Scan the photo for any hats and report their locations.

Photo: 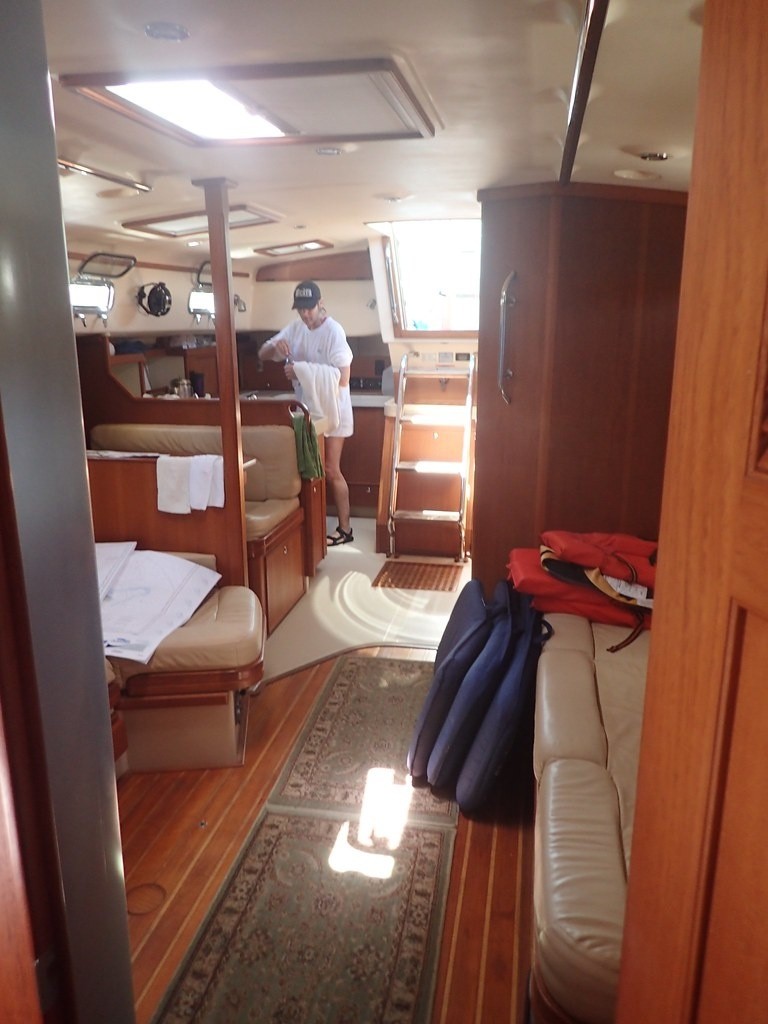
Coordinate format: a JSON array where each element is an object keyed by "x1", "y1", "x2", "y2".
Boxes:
[{"x1": 291, "y1": 280, "x2": 321, "y2": 310}]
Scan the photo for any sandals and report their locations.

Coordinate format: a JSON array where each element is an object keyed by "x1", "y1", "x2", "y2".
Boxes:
[{"x1": 326, "y1": 526, "x2": 354, "y2": 546}]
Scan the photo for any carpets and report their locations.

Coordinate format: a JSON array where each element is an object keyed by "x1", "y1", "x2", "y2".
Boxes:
[{"x1": 151, "y1": 654, "x2": 480, "y2": 1024}]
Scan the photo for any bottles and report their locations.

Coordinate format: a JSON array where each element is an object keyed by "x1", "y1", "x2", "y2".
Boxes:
[{"x1": 285, "y1": 355, "x2": 294, "y2": 365}]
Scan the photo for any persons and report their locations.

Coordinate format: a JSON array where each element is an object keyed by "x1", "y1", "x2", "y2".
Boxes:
[{"x1": 257, "y1": 281, "x2": 353, "y2": 547}]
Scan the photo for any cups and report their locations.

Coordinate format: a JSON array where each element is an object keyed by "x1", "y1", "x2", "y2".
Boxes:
[{"x1": 178, "y1": 379, "x2": 191, "y2": 398}]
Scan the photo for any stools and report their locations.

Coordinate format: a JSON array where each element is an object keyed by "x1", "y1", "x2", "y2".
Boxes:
[{"x1": 93, "y1": 552, "x2": 266, "y2": 767}]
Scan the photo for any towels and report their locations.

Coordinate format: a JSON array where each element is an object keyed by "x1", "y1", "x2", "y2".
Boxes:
[
  {"x1": 290, "y1": 414, "x2": 326, "y2": 481},
  {"x1": 156, "y1": 455, "x2": 225, "y2": 515},
  {"x1": 292, "y1": 360, "x2": 341, "y2": 437},
  {"x1": 95, "y1": 542, "x2": 223, "y2": 665}
]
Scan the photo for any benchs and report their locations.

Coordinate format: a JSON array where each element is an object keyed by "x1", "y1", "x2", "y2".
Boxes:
[
  {"x1": 519, "y1": 604, "x2": 648, "y2": 1024},
  {"x1": 88, "y1": 422, "x2": 306, "y2": 640}
]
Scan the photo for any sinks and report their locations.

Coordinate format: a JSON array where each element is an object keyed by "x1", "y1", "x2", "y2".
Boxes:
[{"x1": 293, "y1": 414, "x2": 323, "y2": 424}]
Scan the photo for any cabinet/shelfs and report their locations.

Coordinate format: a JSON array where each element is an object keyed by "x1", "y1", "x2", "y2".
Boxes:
[{"x1": 324, "y1": 406, "x2": 384, "y2": 506}]
